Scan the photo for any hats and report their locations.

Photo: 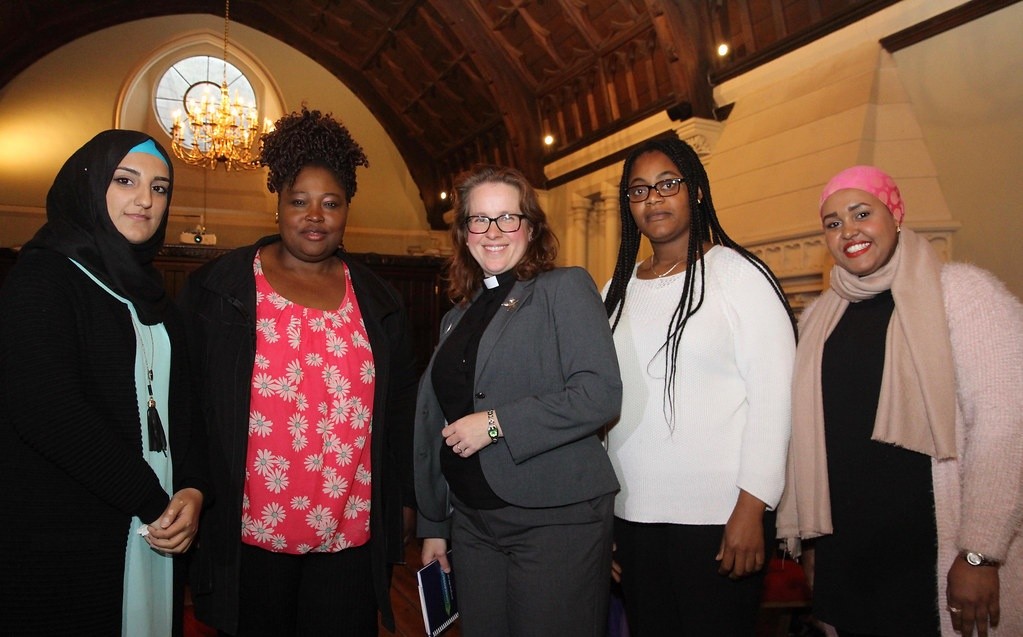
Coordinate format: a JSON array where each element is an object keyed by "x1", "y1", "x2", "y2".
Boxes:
[{"x1": 820, "y1": 165, "x2": 909, "y2": 223}]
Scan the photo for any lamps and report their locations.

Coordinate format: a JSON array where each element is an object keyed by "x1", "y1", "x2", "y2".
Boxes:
[{"x1": 169, "y1": 1, "x2": 276, "y2": 174}]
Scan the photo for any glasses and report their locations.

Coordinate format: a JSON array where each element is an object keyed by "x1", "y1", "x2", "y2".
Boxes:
[
  {"x1": 466, "y1": 214, "x2": 528, "y2": 235},
  {"x1": 623, "y1": 178, "x2": 689, "y2": 203}
]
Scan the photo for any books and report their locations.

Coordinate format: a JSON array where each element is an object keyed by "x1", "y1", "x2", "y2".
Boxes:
[{"x1": 416, "y1": 548, "x2": 460, "y2": 636}]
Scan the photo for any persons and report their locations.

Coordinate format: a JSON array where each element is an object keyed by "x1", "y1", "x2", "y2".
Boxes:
[
  {"x1": 0, "y1": 128, "x2": 216, "y2": 637},
  {"x1": 178, "y1": 102, "x2": 421, "y2": 635},
  {"x1": 598, "y1": 137, "x2": 796, "y2": 637},
  {"x1": 410, "y1": 164, "x2": 625, "y2": 636},
  {"x1": 793, "y1": 165, "x2": 1022, "y2": 637}
]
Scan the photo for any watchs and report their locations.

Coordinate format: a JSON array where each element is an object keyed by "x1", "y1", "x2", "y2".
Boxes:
[{"x1": 959, "y1": 549, "x2": 1001, "y2": 567}]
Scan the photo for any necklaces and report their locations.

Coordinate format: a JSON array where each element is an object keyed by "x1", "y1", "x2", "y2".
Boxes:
[
  {"x1": 647, "y1": 249, "x2": 689, "y2": 279},
  {"x1": 129, "y1": 303, "x2": 168, "y2": 455}
]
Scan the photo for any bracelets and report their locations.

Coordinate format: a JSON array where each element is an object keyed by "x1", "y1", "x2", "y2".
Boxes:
[{"x1": 486, "y1": 410, "x2": 498, "y2": 443}]
